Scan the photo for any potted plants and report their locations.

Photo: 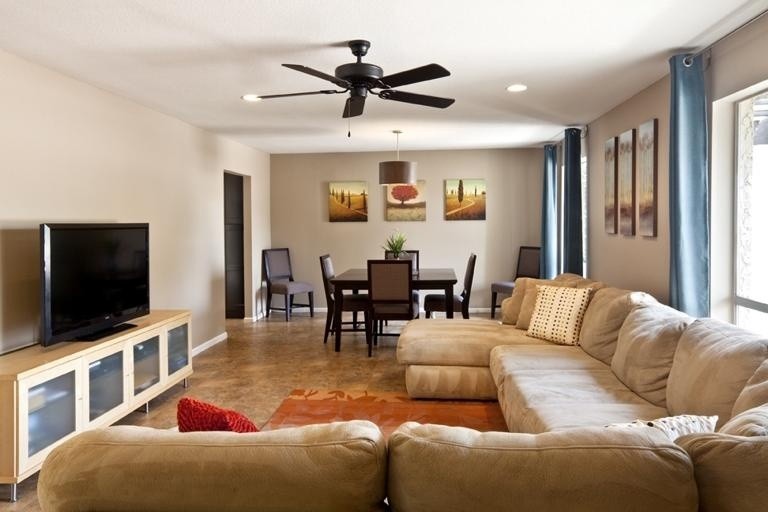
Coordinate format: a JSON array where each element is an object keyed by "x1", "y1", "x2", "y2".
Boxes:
[
  {"x1": 382, "y1": 226, "x2": 408, "y2": 260},
  {"x1": 490, "y1": 246, "x2": 540, "y2": 320}
]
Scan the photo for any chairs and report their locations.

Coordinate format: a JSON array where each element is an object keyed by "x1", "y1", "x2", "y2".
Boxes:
[
  {"x1": 319, "y1": 250, "x2": 477, "y2": 357},
  {"x1": 262, "y1": 248, "x2": 314, "y2": 322}
]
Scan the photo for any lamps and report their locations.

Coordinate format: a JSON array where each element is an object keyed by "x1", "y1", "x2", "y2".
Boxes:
[{"x1": 378, "y1": 130, "x2": 416, "y2": 186}]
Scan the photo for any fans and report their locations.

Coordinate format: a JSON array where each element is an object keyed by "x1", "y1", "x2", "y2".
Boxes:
[{"x1": 257, "y1": 40, "x2": 455, "y2": 119}]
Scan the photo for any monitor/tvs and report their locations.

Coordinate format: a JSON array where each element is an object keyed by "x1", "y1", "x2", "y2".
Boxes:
[{"x1": 40, "y1": 224, "x2": 149, "y2": 346}]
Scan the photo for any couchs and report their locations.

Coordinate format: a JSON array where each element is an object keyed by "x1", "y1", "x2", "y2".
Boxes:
[
  {"x1": 37, "y1": 424, "x2": 768, "y2": 512},
  {"x1": 397, "y1": 273, "x2": 611, "y2": 398},
  {"x1": 489, "y1": 287, "x2": 768, "y2": 435}
]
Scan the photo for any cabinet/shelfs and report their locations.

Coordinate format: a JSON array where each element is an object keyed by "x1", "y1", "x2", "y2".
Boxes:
[{"x1": 0, "y1": 308, "x2": 193, "y2": 502}]
